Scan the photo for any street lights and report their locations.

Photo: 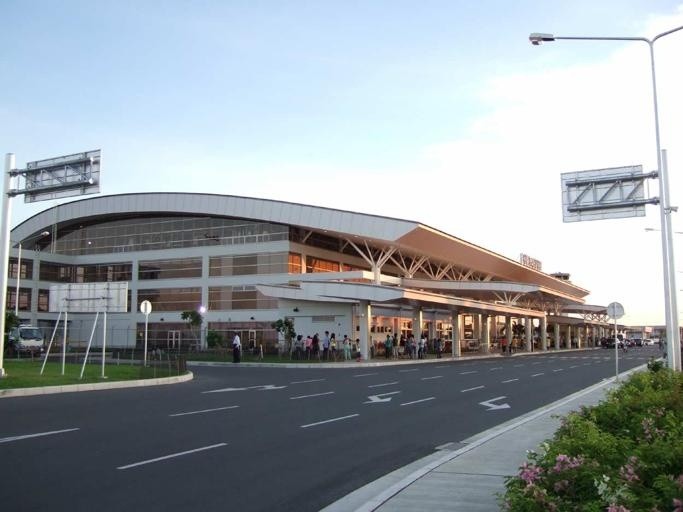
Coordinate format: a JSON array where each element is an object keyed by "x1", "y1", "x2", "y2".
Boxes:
[
  {"x1": 14, "y1": 231, "x2": 50, "y2": 319},
  {"x1": 529, "y1": 28, "x2": 676, "y2": 367}
]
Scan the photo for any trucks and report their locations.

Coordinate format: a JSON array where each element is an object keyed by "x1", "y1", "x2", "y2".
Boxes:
[{"x1": 5, "y1": 324, "x2": 44, "y2": 358}]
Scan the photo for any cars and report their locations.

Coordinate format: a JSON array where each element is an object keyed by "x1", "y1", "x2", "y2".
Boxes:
[{"x1": 600, "y1": 332, "x2": 661, "y2": 349}]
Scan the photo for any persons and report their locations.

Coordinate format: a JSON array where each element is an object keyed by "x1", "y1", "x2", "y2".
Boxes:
[
  {"x1": 384, "y1": 334, "x2": 392, "y2": 360},
  {"x1": 230, "y1": 330, "x2": 241, "y2": 364},
  {"x1": 623, "y1": 340, "x2": 628, "y2": 353},
  {"x1": 498, "y1": 336, "x2": 507, "y2": 353},
  {"x1": 354, "y1": 338, "x2": 362, "y2": 362},
  {"x1": 399, "y1": 334, "x2": 428, "y2": 360},
  {"x1": 391, "y1": 332, "x2": 399, "y2": 361},
  {"x1": 510, "y1": 335, "x2": 517, "y2": 353},
  {"x1": 435, "y1": 339, "x2": 442, "y2": 358},
  {"x1": 286, "y1": 330, "x2": 352, "y2": 362}
]
